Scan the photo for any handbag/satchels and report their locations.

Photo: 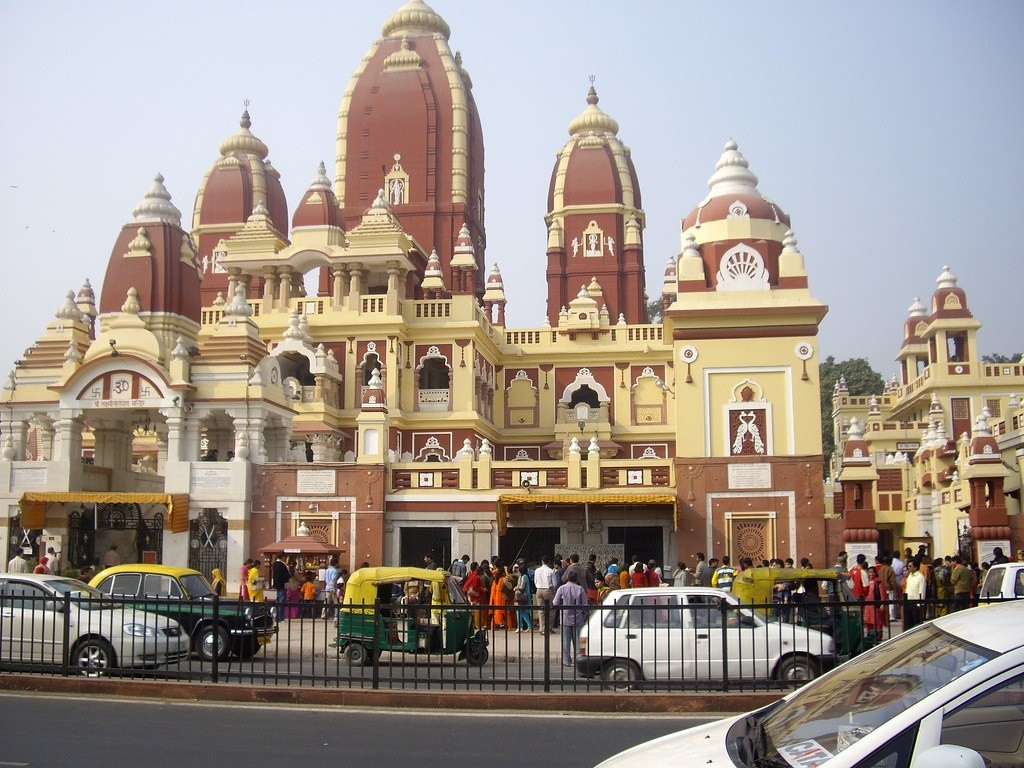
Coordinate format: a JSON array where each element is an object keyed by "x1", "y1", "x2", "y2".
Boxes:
[{"x1": 514, "y1": 589, "x2": 528, "y2": 602}]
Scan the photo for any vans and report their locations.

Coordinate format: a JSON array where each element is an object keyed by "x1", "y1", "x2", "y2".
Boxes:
[{"x1": 978, "y1": 562, "x2": 1024, "y2": 607}]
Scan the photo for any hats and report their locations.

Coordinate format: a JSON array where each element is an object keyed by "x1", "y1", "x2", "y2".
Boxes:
[{"x1": 40, "y1": 556, "x2": 48, "y2": 564}]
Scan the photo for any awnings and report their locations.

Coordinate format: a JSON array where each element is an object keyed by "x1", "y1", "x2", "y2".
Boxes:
[
  {"x1": 18, "y1": 491, "x2": 190, "y2": 533},
  {"x1": 494, "y1": 493, "x2": 681, "y2": 536}
]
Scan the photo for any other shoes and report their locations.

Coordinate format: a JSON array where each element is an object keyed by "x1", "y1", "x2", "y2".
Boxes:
[
  {"x1": 539, "y1": 631, "x2": 545, "y2": 635},
  {"x1": 548, "y1": 628, "x2": 556, "y2": 633},
  {"x1": 524, "y1": 629, "x2": 532, "y2": 633},
  {"x1": 514, "y1": 628, "x2": 523, "y2": 633}
]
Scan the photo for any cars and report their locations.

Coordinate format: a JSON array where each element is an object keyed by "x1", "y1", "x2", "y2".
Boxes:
[
  {"x1": 0, "y1": 571, "x2": 192, "y2": 677},
  {"x1": 570, "y1": 586, "x2": 836, "y2": 692},
  {"x1": 591, "y1": 599, "x2": 1024, "y2": 768},
  {"x1": 45, "y1": 563, "x2": 280, "y2": 660}
]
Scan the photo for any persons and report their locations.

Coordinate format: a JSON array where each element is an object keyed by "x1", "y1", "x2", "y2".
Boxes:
[
  {"x1": 604, "y1": 235, "x2": 615, "y2": 256},
  {"x1": 589, "y1": 234, "x2": 600, "y2": 255},
  {"x1": 33, "y1": 547, "x2": 59, "y2": 575},
  {"x1": 102, "y1": 543, "x2": 121, "y2": 571},
  {"x1": 79, "y1": 566, "x2": 93, "y2": 583},
  {"x1": 226, "y1": 451, "x2": 234, "y2": 460},
  {"x1": 553, "y1": 570, "x2": 589, "y2": 667},
  {"x1": 571, "y1": 237, "x2": 583, "y2": 258},
  {"x1": 424, "y1": 545, "x2": 1012, "y2": 638},
  {"x1": 391, "y1": 178, "x2": 402, "y2": 205},
  {"x1": 211, "y1": 568, "x2": 226, "y2": 597},
  {"x1": 203, "y1": 449, "x2": 218, "y2": 461},
  {"x1": 202, "y1": 254, "x2": 212, "y2": 274},
  {"x1": 8, "y1": 547, "x2": 29, "y2": 573},
  {"x1": 239, "y1": 552, "x2": 370, "y2": 621}
]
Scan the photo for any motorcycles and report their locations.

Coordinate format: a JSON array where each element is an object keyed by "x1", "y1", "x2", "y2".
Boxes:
[
  {"x1": 328, "y1": 564, "x2": 490, "y2": 667},
  {"x1": 729, "y1": 567, "x2": 869, "y2": 657}
]
